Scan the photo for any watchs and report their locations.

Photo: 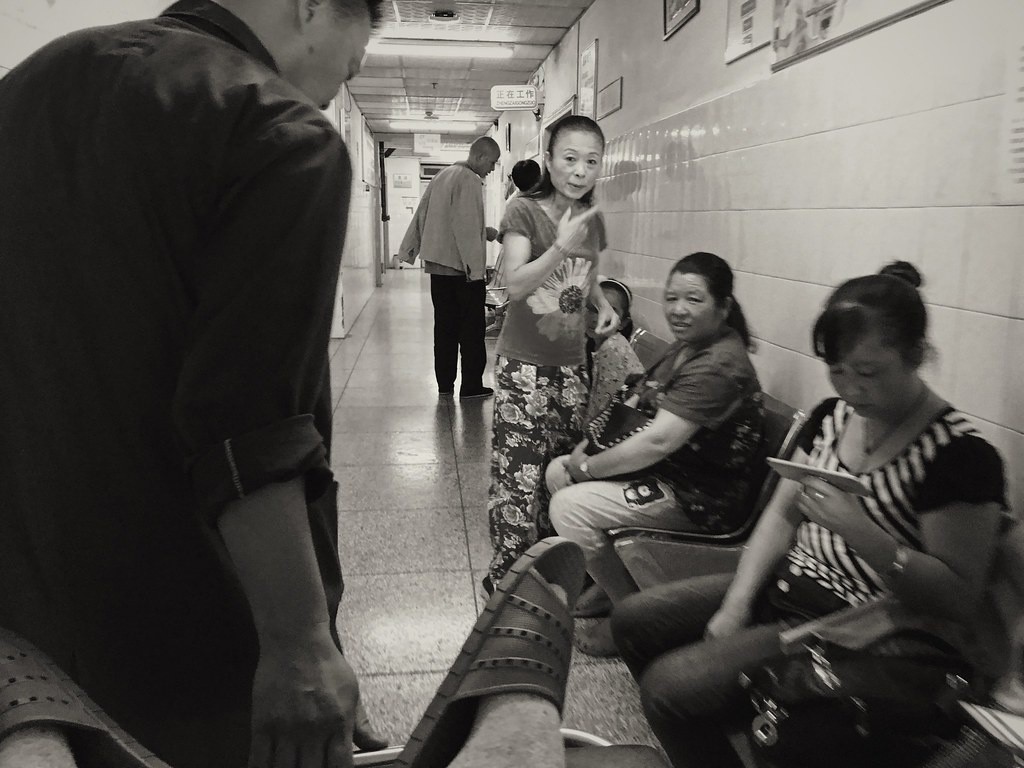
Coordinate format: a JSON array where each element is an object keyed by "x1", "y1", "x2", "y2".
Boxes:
[
  {"x1": 874, "y1": 539, "x2": 912, "y2": 581},
  {"x1": 581, "y1": 455, "x2": 595, "y2": 480}
]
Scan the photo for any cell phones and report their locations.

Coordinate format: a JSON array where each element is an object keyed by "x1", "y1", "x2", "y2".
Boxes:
[{"x1": 765, "y1": 456, "x2": 874, "y2": 497}]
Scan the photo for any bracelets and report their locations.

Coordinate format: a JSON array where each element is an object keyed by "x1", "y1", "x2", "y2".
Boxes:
[{"x1": 549, "y1": 239, "x2": 574, "y2": 259}]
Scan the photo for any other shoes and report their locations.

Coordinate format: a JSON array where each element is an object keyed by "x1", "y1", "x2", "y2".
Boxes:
[
  {"x1": 572, "y1": 618, "x2": 620, "y2": 659},
  {"x1": 571, "y1": 584, "x2": 614, "y2": 619},
  {"x1": 438, "y1": 388, "x2": 454, "y2": 394},
  {"x1": 460, "y1": 386, "x2": 493, "y2": 399}
]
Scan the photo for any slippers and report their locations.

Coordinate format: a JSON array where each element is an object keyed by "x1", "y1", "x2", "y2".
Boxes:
[
  {"x1": 394, "y1": 535, "x2": 586, "y2": 768},
  {"x1": 1, "y1": 625, "x2": 175, "y2": 768}
]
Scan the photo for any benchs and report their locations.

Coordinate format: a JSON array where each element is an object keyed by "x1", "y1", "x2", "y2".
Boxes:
[
  {"x1": 478, "y1": 247, "x2": 512, "y2": 334},
  {"x1": 612, "y1": 460, "x2": 1022, "y2": 768},
  {"x1": 526, "y1": 330, "x2": 805, "y2": 543}
]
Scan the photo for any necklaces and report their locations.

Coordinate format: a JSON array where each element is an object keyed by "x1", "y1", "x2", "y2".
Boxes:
[{"x1": 862, "y1": 380, "x2": 928, "y2": 457}]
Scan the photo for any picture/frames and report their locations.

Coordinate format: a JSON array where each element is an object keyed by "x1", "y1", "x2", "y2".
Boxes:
[
  {"x1": 576, "y1": 37, "x2": 598, "y2": 126},
  {"x1": 596, "y1": 77, "x2": 623, "y2": 123},
  {"x1": 722, "y1": 1, "x2": 774, "y2": 65},
  {"x1": 661, "y1": 1, "x2": 699, "y2": 42},
  {"x1": 769, "y1": 0, "x2": 952, "y2": 72}
]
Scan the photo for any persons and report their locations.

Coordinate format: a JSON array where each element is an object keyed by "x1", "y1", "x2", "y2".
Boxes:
[
  {"x1": 395, "y1": 136, "x2": 501, "y2": 399},
  {"x1": 496, "y1": 158, "x2": 541, "y2": 244},
  {"x1": 0, "y1": 0, "x2": 388, "y2": 768},
  {"x1": 481, "y1": 115, "x2": 624, "y2": 600},
  {"x1": 0, "y1": 535, "x2": 585, "y2": 767},
  {"x1": 581, "y1": 278, "x2": 648, "y2": 439},
  {"x1": 544, "y1": 251, "x2": 767, "y2": 658},
  {"x1": 606, "y1": 259, "x2": 1010, "y2": 767}
]
